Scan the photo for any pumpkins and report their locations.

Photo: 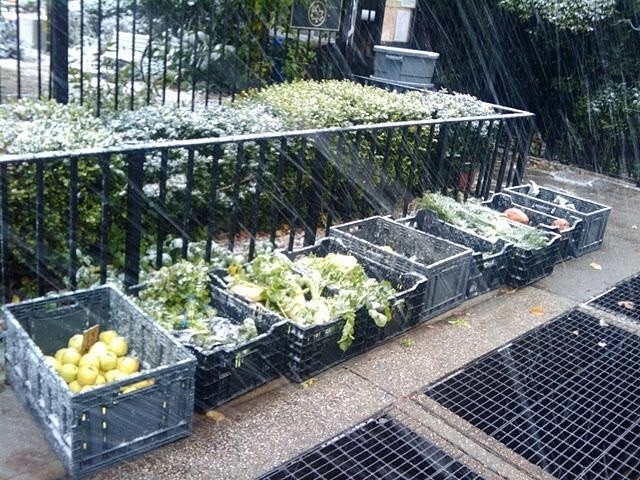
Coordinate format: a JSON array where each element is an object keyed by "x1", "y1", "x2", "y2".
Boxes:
[
  {"x1": 551, "y1": 218, "x2": 571, "y2": 230},
  {"x1": 504, "y1": 207, "x2": 529, "y2": 225}
]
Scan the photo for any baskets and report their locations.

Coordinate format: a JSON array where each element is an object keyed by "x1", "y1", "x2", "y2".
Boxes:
[
  {"x1": 329, "y1": 184, "x2": 612, "y2": 321},
  {"x1": 126, "y1": 279, "x2": 289, "y2": 414},
  {"x1": 0, "y1": 284, "x2": 196, "y2": 478},
  {"x1": 211, "y1": 237, "x2": 428, "y2": 383}
]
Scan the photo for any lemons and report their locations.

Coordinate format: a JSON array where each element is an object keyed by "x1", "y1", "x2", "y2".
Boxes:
[{"x1": 44, "y1": 330, "x2": 155, "y2": 396}]
